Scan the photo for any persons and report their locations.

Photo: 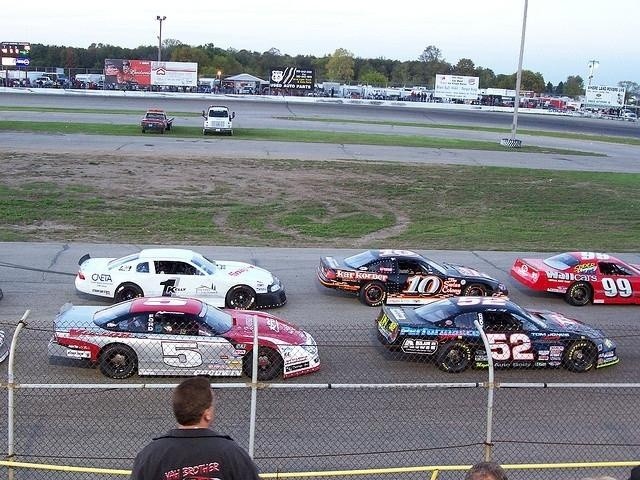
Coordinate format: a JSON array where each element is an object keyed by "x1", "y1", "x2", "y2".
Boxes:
[
  {"x1": 116, "y1": 60, "x2": 134, "y2": 83},
  {"x1": 476, "y1": 95, "x2": 500, "y2": 107},
  {"x1": 371, "y1": 88, "x2": 433, "y2": 103},
  {"x1": 464, "y1": 461, "x2": 508, "y2": 480},
  {"x1": 128, "y1": 377, "x2": 260, "y2": 480}
]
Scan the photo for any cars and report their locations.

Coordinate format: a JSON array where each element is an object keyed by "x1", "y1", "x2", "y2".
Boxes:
[
  {"x1": 0, "y1": 74, "x2": 103, "y2": 87},
  {"x1": 541, "y1": 104, "x2": 637, "y2": 121}
]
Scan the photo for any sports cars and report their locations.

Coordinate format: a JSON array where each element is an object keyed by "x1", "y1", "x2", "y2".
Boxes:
[
  {"x1": 75, "y1": 248, "x2": 287, "y2": 310},
  {"x1": 511, "y1": 252, "x2": 640, "y2": 307},
  {"x1": 376, "y1": 296, "x2": 619, "y2": 374},
  {"x1": 316, "y1": 250, "x2": 508, "y2": 307},
  {"x1": 48, "y1": 296, "x2": 320, "y2": 379}
]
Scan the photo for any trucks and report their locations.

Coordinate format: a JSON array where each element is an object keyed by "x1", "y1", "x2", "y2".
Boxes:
[
  {"x1": 202, "y1": 106, "x2": 235, "y2": 136},
  {"x1": 141, "y1": 110, "x2": 174, "y2": 134}
]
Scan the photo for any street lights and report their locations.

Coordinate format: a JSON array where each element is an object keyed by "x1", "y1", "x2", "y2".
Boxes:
[{"x1": 156, "y1": 15, "x2": 166, "y2": 61}]
